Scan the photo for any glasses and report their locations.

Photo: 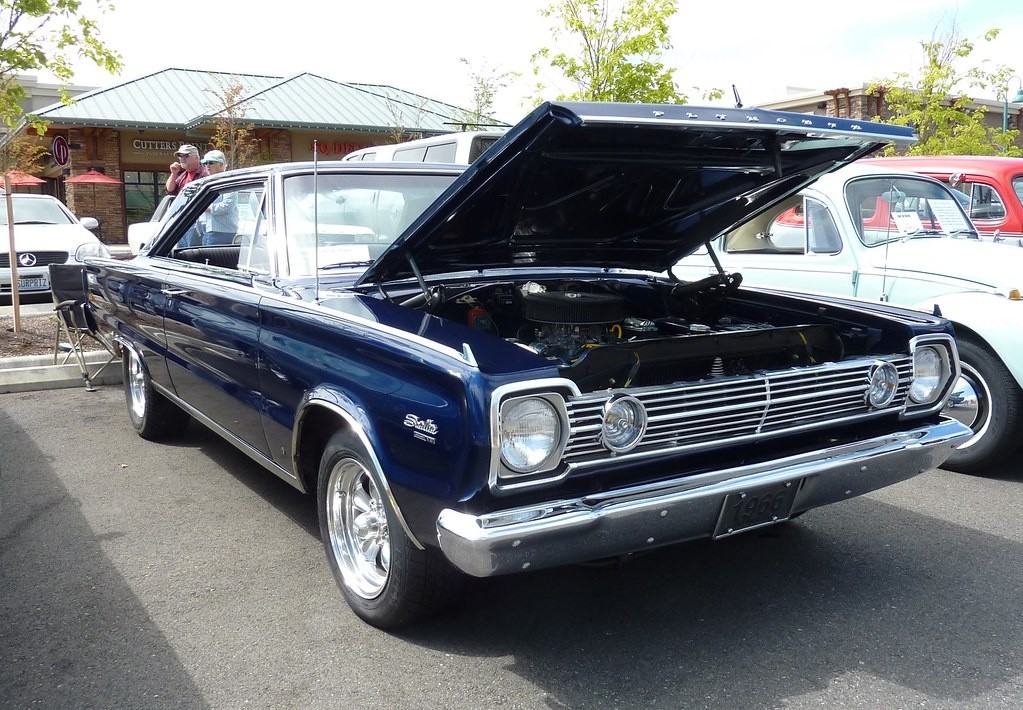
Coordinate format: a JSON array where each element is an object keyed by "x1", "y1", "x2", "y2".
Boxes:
[
  {"x1": 205, "y1": 161, "x2": 217, "y2": 165},
  {"x1": 178, "y1": 154, "x2": 192, "y2": 159}
]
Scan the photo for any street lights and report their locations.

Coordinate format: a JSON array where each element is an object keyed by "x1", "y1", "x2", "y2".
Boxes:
[{"x1": 1002, "y1": 75, "x2": 1023, "y2": 153}]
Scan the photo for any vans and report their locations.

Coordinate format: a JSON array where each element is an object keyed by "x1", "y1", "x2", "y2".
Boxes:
[
  {"x1": 336, "y1": 131, "x2": 504, "y2": 165},
  {"x1": 769, "y1": 155, "x2": 1022, "y2": 231}
]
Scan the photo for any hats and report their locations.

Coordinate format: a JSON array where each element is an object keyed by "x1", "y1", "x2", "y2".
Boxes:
[
  {"x1": 174, "y1": 145, "x2": 198, "y2": 156},
  {"x1": 200, "y1": 150, "x2": 226, "y2": 163}
]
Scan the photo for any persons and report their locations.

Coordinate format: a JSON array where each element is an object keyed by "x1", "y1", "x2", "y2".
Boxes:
[{"x1": 164, "y1": 144, "x2": 241, "y2": 249}]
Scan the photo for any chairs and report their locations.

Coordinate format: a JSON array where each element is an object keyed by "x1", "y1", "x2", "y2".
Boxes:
[{"x1": 47, "y1": 261, "x2": 122, "y2": 393}]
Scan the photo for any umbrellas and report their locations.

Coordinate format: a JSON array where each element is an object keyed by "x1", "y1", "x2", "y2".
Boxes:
[
  {"x1": 0, "y1": 166, "x2": 47, "y2": 193},
  {"x1": 63, "y1": 168, "x2": 124, "y2": 237}
]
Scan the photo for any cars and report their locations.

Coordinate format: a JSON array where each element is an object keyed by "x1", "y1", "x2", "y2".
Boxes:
[
  {"x1": 82, "y1": 100, "x2": 973, "y2": 631},
  {"x1": 614, "y1": 159, "x2": 1023, "y2": 469},
  {"x1": 0, "y1": 193, "x2": 113, "y2": 296},
  {"x1": 127, "y1": 192, "x2": 375, "y2": 256}
]
